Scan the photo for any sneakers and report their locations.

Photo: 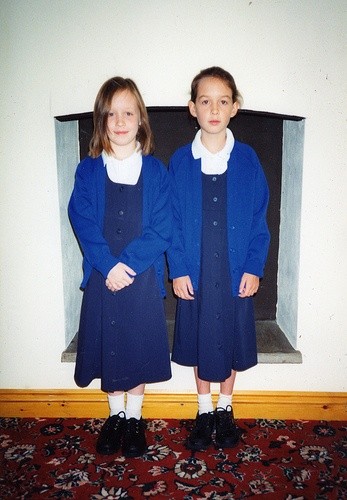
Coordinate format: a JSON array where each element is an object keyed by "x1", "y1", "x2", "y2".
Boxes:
[
  {"x1": 122, "y1": 416, "x2": 148, "y2": 457},
  {"x1": 96, "y1": 411, "x2": 126, "y2": 455},
  {"x1": 212, "y1": 405, "x2": 240, "y2": 448},
  {"x1": 185, "y1": 409, "x2": 217, "y2": 451}
]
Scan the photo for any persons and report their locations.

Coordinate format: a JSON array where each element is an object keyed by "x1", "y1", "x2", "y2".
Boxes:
[
  {"x1": 165, "y1": 66, "x2": 270, "y2": 450},
  {"x1": 68, "y1": 76, "x2": 171, "y2": 458}
]
